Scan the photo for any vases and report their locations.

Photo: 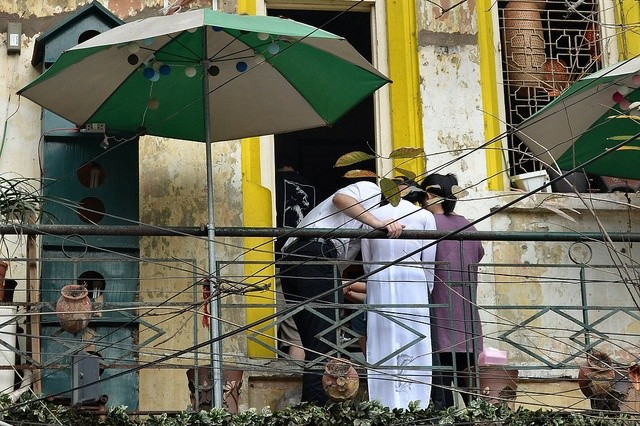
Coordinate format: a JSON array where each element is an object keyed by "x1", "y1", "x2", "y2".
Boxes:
[
  {"x1": 576, "y1": 351, "x2": 616, "y2": 399},
  {"x1": 320, "y1": 355, "x2": 358, "y2": 403},
  {"x1": 185, "y1": 361, "x2": 243, "y2": 417},
  {"x1": 511, "y1": 169, "x2": 548, "y2": 192},
  {"x1": 2, "y1": 275, "x2": 20, "y2": 303},
  {"x1": 547, "y1": 170, "x2": 590, "y2": 193},
  {"x1": 55, "y1": 282, "x2": 94, "y2": 335}
]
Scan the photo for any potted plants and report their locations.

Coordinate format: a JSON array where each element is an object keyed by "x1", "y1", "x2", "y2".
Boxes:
[{"x1": 0, "y1": 168, "x2": 98, "y2": 303}]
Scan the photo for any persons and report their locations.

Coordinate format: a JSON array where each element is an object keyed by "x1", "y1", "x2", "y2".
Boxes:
[
  {"x1": 360, "y1": 179, "x2": 437, "y2": 414},
  {"x1": 275, "y1": 157, "x2": 319, "y2": 360},
  {"x1": 340, "y1": 279, "x2": 367, "y2": 353},
  {"x1": 279, "y1": 178, "x2": 403, "y2": 408},
  {"x1": 419, "y1": 173, "x2": 484, "y2": 411}
]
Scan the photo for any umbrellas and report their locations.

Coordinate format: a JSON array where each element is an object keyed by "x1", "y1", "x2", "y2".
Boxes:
[
  {"x1": 508, "y1": 56, "x2": 639, "y2": 182},
  {"x1": 15, "y1": 8, "x2": 395, "y2": 413}
]
